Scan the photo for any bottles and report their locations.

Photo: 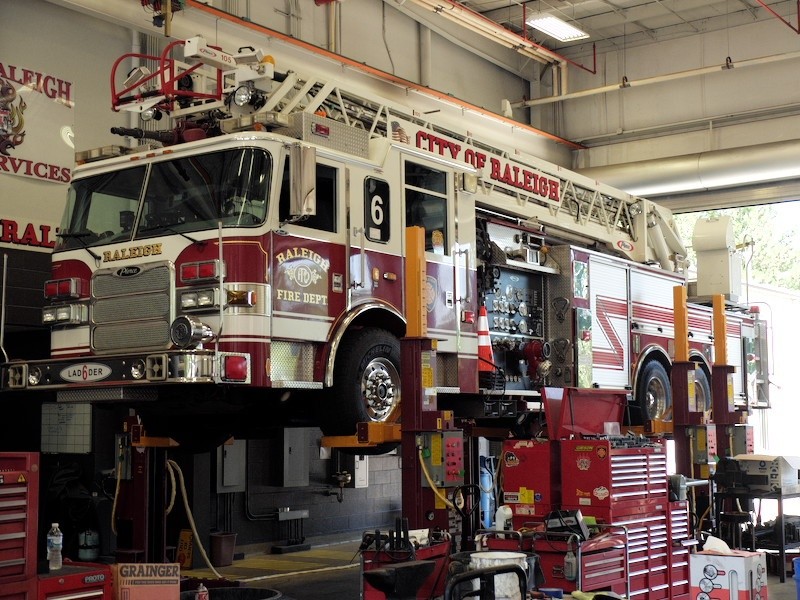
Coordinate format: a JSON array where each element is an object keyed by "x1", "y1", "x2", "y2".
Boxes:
[
  {"x1": 496, "y1": 504, "x2": 513, "y2": 540},
  {"x1": 46, "y1": 524, "x2": 62, "y2": 570}
]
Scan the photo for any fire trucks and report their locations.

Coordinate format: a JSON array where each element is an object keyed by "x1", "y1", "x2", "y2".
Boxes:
[{"x1": 0, "y1": 33, "x2": 800, "y2": 599}]
